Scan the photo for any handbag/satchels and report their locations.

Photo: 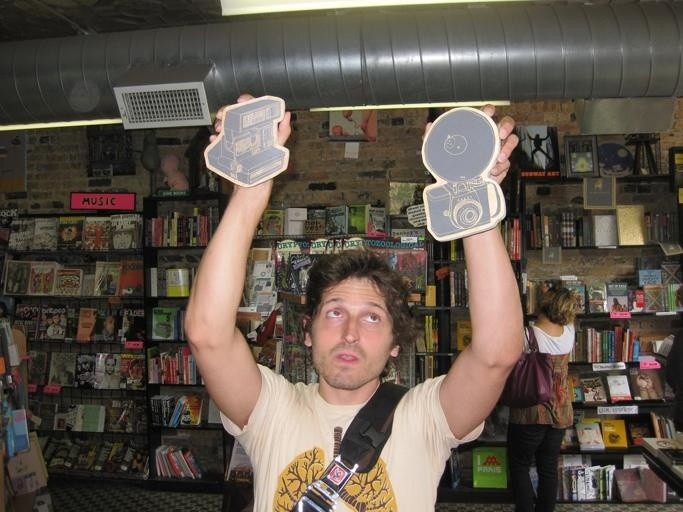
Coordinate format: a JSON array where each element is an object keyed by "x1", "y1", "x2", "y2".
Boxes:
[{"x1": 496, "y1": 322, "x2": 555, "y2": 409}]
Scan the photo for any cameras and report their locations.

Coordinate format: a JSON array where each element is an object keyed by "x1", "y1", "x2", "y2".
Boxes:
[{"x1": 427, "y1": 178, "x2": 491, "y2": 237}]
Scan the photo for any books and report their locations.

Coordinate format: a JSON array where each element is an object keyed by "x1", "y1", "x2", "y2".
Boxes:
[
  {"x1": 240, "y1": 203, "x2": 429, "y2": 310},
  {"x1": 447, "y1": 447, "x2": 508, "y2": 490},
  {"x1": 556, "y1": 407, "x2": 683, "y2": 504},
  {"x1": 432, "y1": 219, "x2": 522, "y2": 352},
  {"x1": 527, "y1": 204, "x2": 683, "y2": 250},
  {"x1": 516, "y1": 124, "x2": 683, "y2": 203},
  {"x1": 151, "y1": 205, "x2": 221, "y2": 247},
  {"x1": 380, "y1": 314, "x2": 438, "y2": 389},
  {"x1": 237, "y1": 305, "x2": 319, "y2": 386},
  {"x1": 149, "y1": 392, "x2": 204, "y2": 480},
  {"x1": 27, "y1": 350, "x2": 147, "y2": 390},
  {"x1": 147, "y1": 306, "x2": 207, "y2": 386},
  {"x1": 151, "y1": 253, "x2": 205, "y2": 298},
  {"x1": 1, "y1": 296, "x2": 145, "y2": 341},
  {"x1": 567, "y1": 324, "x2": 683, "y2": 403},
  {"x1": 385, "y1": 176, "x2": 430, "y2": 219},
  {"x1": 526, "y1": 255, "x2": 683, "y2": 314},
  {"x1": 0, "y1": 213, "x2": 146, "y2": 297},
  {"x1": 25, "y1": 400, "x2": 150, "y2": 480}
]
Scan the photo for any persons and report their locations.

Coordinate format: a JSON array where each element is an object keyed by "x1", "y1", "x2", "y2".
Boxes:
[{"x1": 176, "y1": 91, "x2": 524, "y2": 510}]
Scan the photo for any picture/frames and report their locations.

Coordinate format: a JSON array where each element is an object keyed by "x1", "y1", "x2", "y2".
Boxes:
[{"x1": 562, "y1": 133, "x2": 600, "y2": 178}]
